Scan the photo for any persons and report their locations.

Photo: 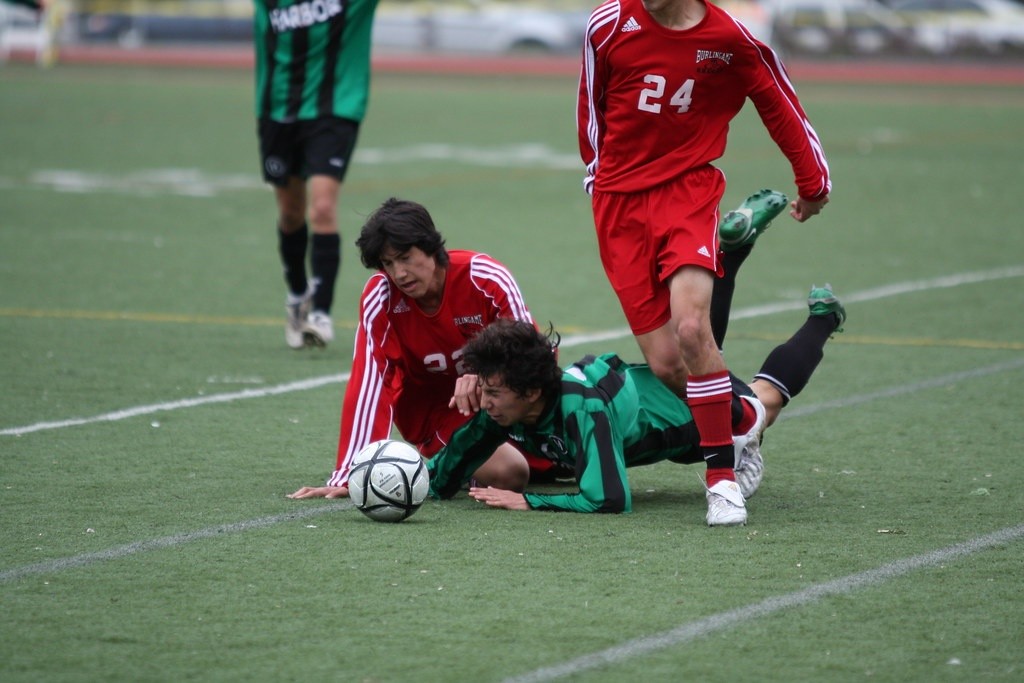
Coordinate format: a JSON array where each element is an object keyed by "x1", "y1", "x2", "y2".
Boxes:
[
  {"x1": 577, "y1": 0, "x2": 831, "y2": 523},
  {"x1": 286, "y1": 198, "x2": 574, "y2": 501},
  {"x1": 253, "y1": 0, "x2": 378, "y2": 349},
  {"x1": 425, "y1": 191, "x2": 846, "y2": 514}
]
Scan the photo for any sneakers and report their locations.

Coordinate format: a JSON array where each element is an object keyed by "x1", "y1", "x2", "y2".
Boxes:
[
  {"x1": 808, "y1": 282, "x2": 847, "y2": 339},
  {"x1": 286, "y1": 299, "x2": 310, "y2": 348},
  {"x1": 732, "y1": 395, "x2": 766, "y2": 498},
  {"x1": 696, "y1": 469, "x2": 748, "y2": 526},
  {"x1": 302, "y1": 311, "x2": 333, "y2": 346},
  {"x1": 719, "y1": 189, "x2": 789, "y2": 253}
]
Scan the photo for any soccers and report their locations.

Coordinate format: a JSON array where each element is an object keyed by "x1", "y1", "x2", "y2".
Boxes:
[{"x1": 345, "y1": 439, "x2": 430, "y2": 525}]
